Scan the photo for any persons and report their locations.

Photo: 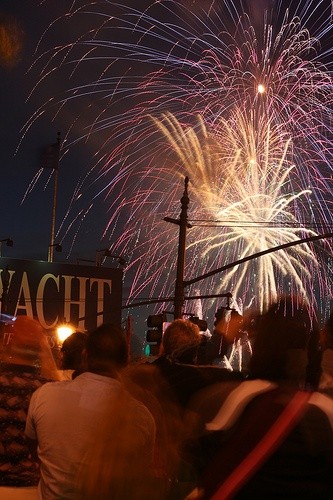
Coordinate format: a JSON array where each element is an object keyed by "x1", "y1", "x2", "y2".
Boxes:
[
  {"x1": 199, "y1": 313, "x2": 242, "y2": 365},
  {"x1": 25, "y1": 323, "x2": 156, "y2": 500},
  {"x1": 69, "y1": 361, "x2": 199, "y2": 500},
  {"x1": 318, "y1": 318, "x2": 333, "y2": 392},
  {"x1": 183, "y1": 293, "x2": 332, "y2": 500},
  {"x1": 59, "y1": 332, "x2": 88, "y2": 369},
  {"x1": 150, "y1": 320, "x2": 245, "y2": 406}
]
